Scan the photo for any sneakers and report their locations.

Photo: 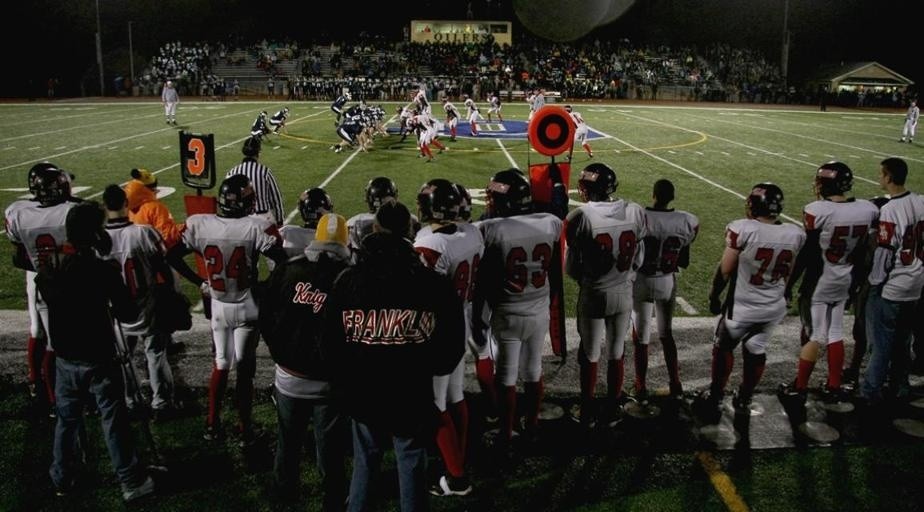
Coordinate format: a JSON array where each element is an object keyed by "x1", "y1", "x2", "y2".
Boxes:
[
  {"x1": 29, "y1": 382, "x2": 46, "y2": 397},
  {"x1": 625, "y1": 395, "x2": 651, "y2": 414},
  {"x1": 238, "y1": 429, "x2": 268, "y2": 444},
  {"x1": 667, "y1": 383, "x2": 684, "y2": 400},
  {"x1": 124, "y1": 467, "x2": 167, "y2": 500},
  {"x1": 734, "y1": 387, "x2": 754, "y2": 408},
  {"x1": 703, "y1": 391, "x2": 726, "y2": 411},
  {"x1": 569, "y1": 404, "x2": 597, "y2": 428},
  {"x1": 151, "y1": 400, "x2": 185, "y2": 415},
  {"x1": 429, "y1": 476, "x2": 472, "y2": 496},
  {"x1": 844, "y1": 381, "x2": 883, "y2": 402},
  {"x1": 605, "y1": 398, "x2": 624, "y2": 415},
  {"x1": 780, "y1": 381, "x2": 807, "y2": 402},
  {"x1": 56, "y1": 477, "x2": 80, "y2": 496},
  {"x1": 203, "y1": 426, "x2": 220, "y2": 438},
  {"x1": 127, "y1": 396, "x2": 152, "y2": 412},
  {"x1": 817, "y1": 381, "x2": 839, "y2": 398},
  {"x1": 885, "y1": 383, "x2": 910, "y2": 398}
]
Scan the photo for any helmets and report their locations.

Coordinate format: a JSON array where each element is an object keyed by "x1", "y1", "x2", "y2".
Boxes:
[
  {"x1": 486, "y1": 169, "x2": 532, "y2": 215},
  {"x1": 416, "y1": 179, "x2": 463, "y2": 222},
  {"x1": 745, "y1": 183, "x2": 784, "y2": 218},
  {"x1": 565, "y1": 104, "x2": 573, "y2": 112},
  {"x1": 284, "y1": 107, "x2": 290, "y2": 113},
  {"x1": 259, "y1": 110, "x2": 268, "y2": 119},
  {"x1": 34, "y1": 168, "x2": 73, "y2": 206},
  {"x1": 439, "y1": 91, "x2": 496, "y2": 102},
  {"x1": 298, "y1": 188, "x2": 336, "y2": 220},
  {"x1": 579, "y1": 162, "x2": 618, "y2": 201},
  {"x1": 394, "y1": 90, "x2": 420, "y2": 127},
  {"x1": 526, "y1": 87, "x2": 540, "y2": 97},
  {"x1": 344, "y1": 92, "x2": 353, "y2": 100},
  {"x1": 359, "y1": 100, "x2": 386, "y2": 129},
  {"x1": 366, "y1": 177, "x2": 399, "y2": 213},
  {"x1": 815, "y1": 161, "x2": 854, "y2": 194},
  {"x1": 29, "y1": 163, "x2": 56, "y2": 193},
  {"x1": 218, "y1": 175, "x2": 256, "y2": 214},
  {"x1": 457, "y1": 183, "x2": 472, "y2": 218}
]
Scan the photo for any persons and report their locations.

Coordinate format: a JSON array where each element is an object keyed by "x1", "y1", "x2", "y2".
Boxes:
[
  {"x1": 257, "y1": 213, "x2": 354, "y2": 512},
  {"x1": 440, "y1": 97, "x2": 461, "y2": 142},
  {"x1": 898, "y1": 98, "x2": 920, "y2": 144},
  {"x1": 314, "y1": 200, "x2": 465, "y2": 512},
  {"x1": 122, "y1": 169, "x2": 186, "y2": 249},
  {"x1": 250, "y1": 110, "x2": 270, "y2": 142},
  {"x1": 476, "y1": 163, "x2": 569, "y2": 442},
  {"x1": 486, "y1": 93, "x2": 503, "y2": 123},
  {"x1": 462, "y1": 94, "x2": 480, "y2": 136},
  {"x1": 345, "y1": 176, "x2": 422, "y2": 267},
  {"x1": 33, "y1": 204, "x2": 160, "y2": 502},
  {"x1": 0, "y1": 161, "x2": 58, "y2": 398},
  {"x1": 397, "y1": 86, "x2": 448, "y2": 162},
  {"x1": 563, "y1": 105, "x2": 592, "y2": 159},
  {"x1": 330, "y1": 90, "x2": 389, "y2": 154},
  {"x1": 411, "y1": 179, "x2": 485, "y2": 496},
  {"x1": 278, "y1": 188, "x2": 333, "y2": 258},
  {"x1": 454, "y1": 183, "x2": 499, "y2": 427},
  {"x1": 161, "y1": 81, "x2": 179, "y2": 126},
  {"x1": 565, "y1": 163, "x2": 647, "y2": 429},
  {"x1": 95, "y1": 184, "x2": 172, "y2": 410},
  {"x1": 632, "y1": 180, "x2": 699, "y2": 405},
  {"x1": 224, "y1": 136, "x2": 284, "y2": 227},
  {"x1": 48, "y1": 77, "x2": 55, "y2": 100},
  {"x1": 268, "y1": 107, "x2": 289, "y2": 134},
  {"x1": 778, "y1": 161, "x2": 879, "y2": 405},
  {"x1": 845, "y1": 196, "x2": 890, "y2": 376},
  {"x1": 113, "y1": 30, "x2": 924, "y2": 111},
  {"x1": 164, "y1": 175, "x2": 289, "y2": 448},
  {"x1": 857, "y1": 156, "x2": 923, "y2": 397},
  {"x1": 701, "y1": 181, "x2": 806, "y2": 414},
  {"x1": 533, "y1": 87, "x2": 544, "y2": 111},
  {"x1": 525, "y1": 88, "x2": 534, "y2": 121},
  {"x1": 14, "y1": 169, "x2": 85, "y2": 406}
]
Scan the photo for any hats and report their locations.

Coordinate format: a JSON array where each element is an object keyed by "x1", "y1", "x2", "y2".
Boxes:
[
  {"x1": 376, "y1": 201, "x2": 413, "y2": 234},
  {"x1": 317, "y1": 215, "x2": 349, "y2": 246},
  {"x1": 131, "y1": 168, "x2": 157, "y2": 187},
  {"x1": 242, "y1": 138, "x2": 262, "y2": 154}
]
